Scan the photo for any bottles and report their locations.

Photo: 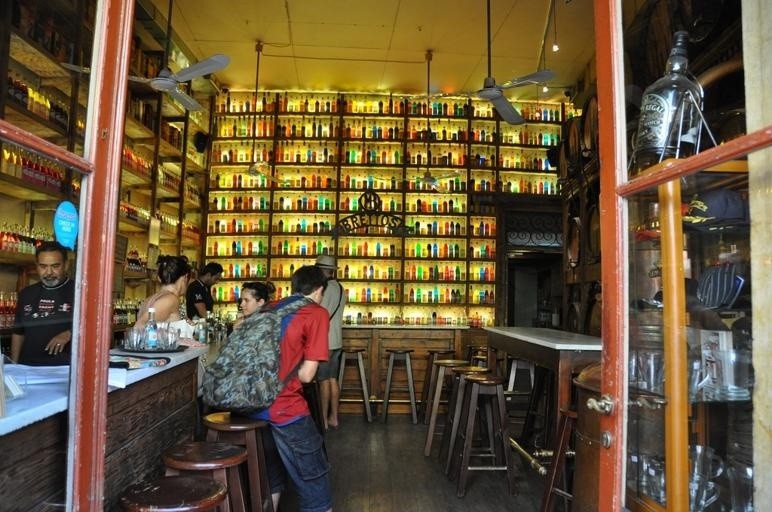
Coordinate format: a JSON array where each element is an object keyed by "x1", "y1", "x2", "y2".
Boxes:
[
  {"x1": 633, "y1": 29, "x2": 706, "y2": 167},
  {"x1": 634, "y1": 202, "x2": 663, "y2": 318},
  {"x1": 198, "y1": 310, "x2": 234, "y2": 345},
  {"x1": 208, "y1": 89, "x2": 578, "y2": 330},
  {"x1": 0, "y1": 18, "x2": 201, "y2": 329}
]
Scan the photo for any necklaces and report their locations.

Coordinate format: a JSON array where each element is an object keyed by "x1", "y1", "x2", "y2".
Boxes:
[{"x1": 42, "y1": 277, "x2": 71, "y2": 290}]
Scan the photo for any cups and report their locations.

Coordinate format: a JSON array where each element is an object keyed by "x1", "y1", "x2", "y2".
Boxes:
[
  {"x1": 123, "y1": 308, "x2": 182, "y2": 351},
  {"x1": 630, "y1": 326, "x2": 752, "y2": 401},
  {"x1": 630, "y1": 446, "x2": 753, "y2": 510}
]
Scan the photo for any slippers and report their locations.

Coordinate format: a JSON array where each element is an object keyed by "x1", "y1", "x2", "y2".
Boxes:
[{"x1": 328, "y1": 425, "x2": 338, "y2": 430}]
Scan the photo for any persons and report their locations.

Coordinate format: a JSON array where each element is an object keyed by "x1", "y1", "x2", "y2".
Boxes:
[
  {"x1": 10, "y1": 241, "x2": 75, "y2": 366},
  {"x1": 315, "y1": 256, "x2": 345, "y2": 432},
  {"x1": 233, "y1": 280, "x2": 276, "y2": 330},
  {"x1": 134, "y1": 255, "x2": 207, "y2": 347},
  {"x1": 247, "y1": 265, "x2": 332, "y2": 511},
  {"x1": 186, "y1": 262, "x2": 223, "y2": 320}
]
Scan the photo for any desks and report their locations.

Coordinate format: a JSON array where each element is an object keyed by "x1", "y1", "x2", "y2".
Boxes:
[{"x1": 479, "y1": 325, "x2": 603, "y2": 486}]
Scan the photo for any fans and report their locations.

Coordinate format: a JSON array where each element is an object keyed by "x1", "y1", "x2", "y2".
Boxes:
[
  {"x1": 414, "y1": 0, "x2": 557, "y2": 125},
  {"x1": 60, "y1": 0, "x2": 230, "y2": 114},
  {"x1": 374, "y1": 48, "x2": 477, "y2": 195},
  {"x1": 231, "y1": 38, "x2": 287, "y2": 183}
]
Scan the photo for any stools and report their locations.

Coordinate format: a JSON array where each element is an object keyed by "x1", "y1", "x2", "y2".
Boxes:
[
  {"x1": 336, "y1": 346, "x2": 417, "y2": 425},
  {"x1": 121, "y1": 376, "x2": 327, "y2": 511},
  {"x1": 417, "y1": 344, "x2": 536, "y2": 498},
  {"x1": 540, "y1": 406, "x2": 577, "y2": 510}
]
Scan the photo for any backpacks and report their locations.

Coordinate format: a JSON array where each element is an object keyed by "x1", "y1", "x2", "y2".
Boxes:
[{"x1": 201, "y1": 298, "x2": 314, "y2": 414}]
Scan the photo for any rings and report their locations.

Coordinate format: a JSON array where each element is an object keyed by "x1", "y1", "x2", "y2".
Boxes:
[{"x1": 56, "y1": 342, "x2": 61, "y2": 347}]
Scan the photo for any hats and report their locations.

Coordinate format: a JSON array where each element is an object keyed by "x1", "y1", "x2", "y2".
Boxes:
[{"x1": 314, "y1": 255, "x2": 339, "y2": 270}]
